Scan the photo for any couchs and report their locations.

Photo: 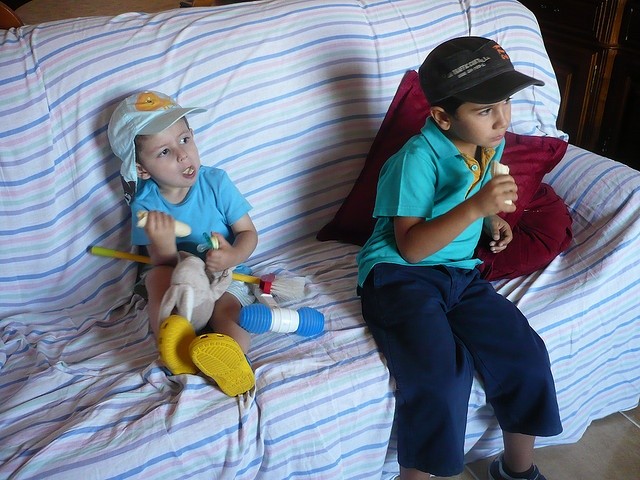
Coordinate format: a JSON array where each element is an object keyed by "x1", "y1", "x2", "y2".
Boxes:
[{"x1": 0, "y1": 0, "x2": 640, "y2": 479}]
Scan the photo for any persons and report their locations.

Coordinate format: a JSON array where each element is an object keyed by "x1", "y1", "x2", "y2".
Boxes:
[
  {"x1": 355, "y1": 36, "x2": 564, "y2": 480},
  {"x1": 107, "y1": 91, "x2": 258, "y2": 397}
]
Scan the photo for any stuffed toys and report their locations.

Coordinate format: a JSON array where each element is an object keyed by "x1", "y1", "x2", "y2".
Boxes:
[{"x1": 158, "y1": 250, "x2": 233, "y2": 335}]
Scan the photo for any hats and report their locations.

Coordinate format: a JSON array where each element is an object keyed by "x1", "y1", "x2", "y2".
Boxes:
[
  {"x1": 418, "y1": 36, "x2": 545, "y2": 104},
  {"x1": 107, "y1": 90, "x2": 208, "y2": 206}
]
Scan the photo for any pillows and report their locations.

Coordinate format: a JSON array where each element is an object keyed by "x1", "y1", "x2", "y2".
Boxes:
[
  {"x1": 486, "y1": 184, "x2": 574, "y2": 283},
  {"x1": 317, "y1": 70, "x2": 568, "y2": 280}
]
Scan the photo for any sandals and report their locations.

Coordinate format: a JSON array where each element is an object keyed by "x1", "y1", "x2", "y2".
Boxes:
[
  {"x1": 188, "y1": 333, "x2": 256, "y2": 397},
  {"x1": 157, "y1": 315, "x2": 201, "y2": 376}
]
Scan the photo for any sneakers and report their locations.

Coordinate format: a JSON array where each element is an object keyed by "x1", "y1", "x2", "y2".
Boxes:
[{"x1": 488, "y1": 451, "x2": 548, "y2": 480}]
